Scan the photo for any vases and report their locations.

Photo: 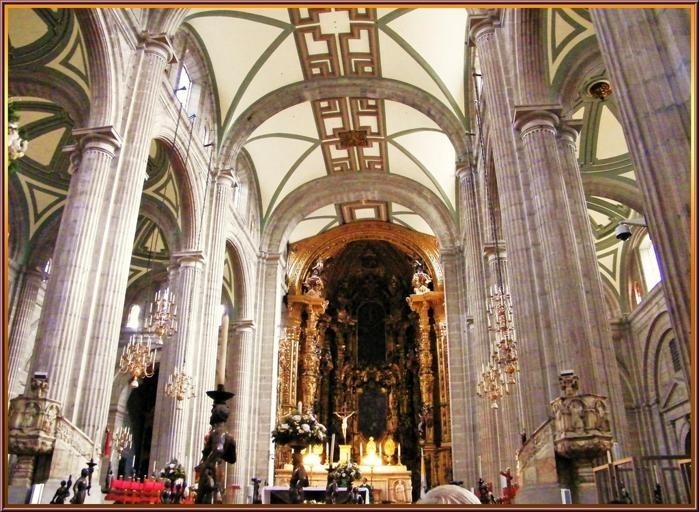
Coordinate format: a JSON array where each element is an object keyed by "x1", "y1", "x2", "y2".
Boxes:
[
  {"x1": 287, "y1": 438, "x2": 309, "y2": 454},
  {"x1": 338, "y1": 480, "x2": 350, "y2": 487}
]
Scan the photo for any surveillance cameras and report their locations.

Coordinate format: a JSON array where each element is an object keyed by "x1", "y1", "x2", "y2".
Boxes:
[{"x1": 615, "y1": 224, "x2": 631, "y2": 241}]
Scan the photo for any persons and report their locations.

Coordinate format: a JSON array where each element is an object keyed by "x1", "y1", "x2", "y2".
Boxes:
[
  {"x1": 159, "y1": 480, "x2": 169, "y2": 503},
  {"x1": 70, "y1": 467, "x2": 92, "y2": 504},
  {"x1": 46, "y1": 479, "x2": 71, "y2": 506},
  {"x1": 288, "y1": 453, "x2": 309, "y2": 504},
  {"x1": 553, "y1": 402, "x2": 564, "y2": 432},
  {"x1": 414, "y1": 484, "x2": 481, "y2": 505},
  {"x1": 351, "y1": 487, "x2": 364, "y2": 505},
  {"x1": 591, "y1": 400, "x2": 610, "y2": 434},
  {"x1": 359, "y1": 477, "x2": 374, "y2": 504},
  {"x1": 171, "y1": 484, "x2": 186, "y2": 504},
  {"x1": 477, "y1": 477, "x2": 497, "y2": 505},
  {"x1": 191, "y1": 401, "x2": 230, "y2": 504},
  {"x1": 569, "y1": 401, "x2": 591, "y2": 433},
  {"x1": 333, "y1": 410, "x2": 355, "y2": 445},
  {"x1": 20, "y1": 403, "x2": 36, "y2": 429},
  {"x1": 620, "y1": 487, "x2": 635, "y2": 504}
]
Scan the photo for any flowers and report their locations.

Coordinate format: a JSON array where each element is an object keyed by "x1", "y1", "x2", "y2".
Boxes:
[
  {"x1": 328, "y1": 460, "x2": 362, "y2": 483},
  {"x1": 271, "y1": 409, "x2": 328, "y2": 447}
]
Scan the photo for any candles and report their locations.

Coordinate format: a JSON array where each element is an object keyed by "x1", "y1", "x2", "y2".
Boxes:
[
  {"x1": 153, "y1": 460, "x2": 156, "y2": 473},
  {"x1": 132, "y1": 454, "x2": 136, "y2": 468},
  {"x1": 298, "y1": 400, "x2": 303, "y2": 412},
  {"x1": 325, "y1": 443, "x2": 330, "y2": 462},
  {"x1": 330, "y1": 433, "x2": 335, "y2": 462},
  {"x1": 421, "y1": 447, "x2": 424, "y2": 456},
  {"x1": 398, "y1": 442, "x2": 401, "y2": 456},
  {"x1": 379, "y1": 442, "x2": 382, "y2": 457},
  {"x1": 360, "y1": 442, "x2": 363, "y2": 456},
  {"x1": 478, "y1": 455, "x2": 482, "y2": 479}
]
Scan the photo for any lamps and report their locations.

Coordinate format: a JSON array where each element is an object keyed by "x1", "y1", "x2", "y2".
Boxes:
[
  {"x1": 164, "y1": 142, "x2": 200, "y2": 401},
  {"x1": 118, "y1": 332, "x2": 158, "y2": 387},
  {"x1": 8, "y1": 94, "x2": 28, "y2": 168},
  {"x1": 112, "y1": 424, "x2": 133, "y2": 461},
  {"x1": 145, "y1": 113, "x2": 198, "y2": 337},
  {"x1": 615, "y1": 221, "x2": 648, "y2": 242},
  {"x1": 485, "y1": 200, "x2": 520, "y2": 387},
  {"x1": 475, "y1": 335, "x2": 506, "y2": 410}
]
